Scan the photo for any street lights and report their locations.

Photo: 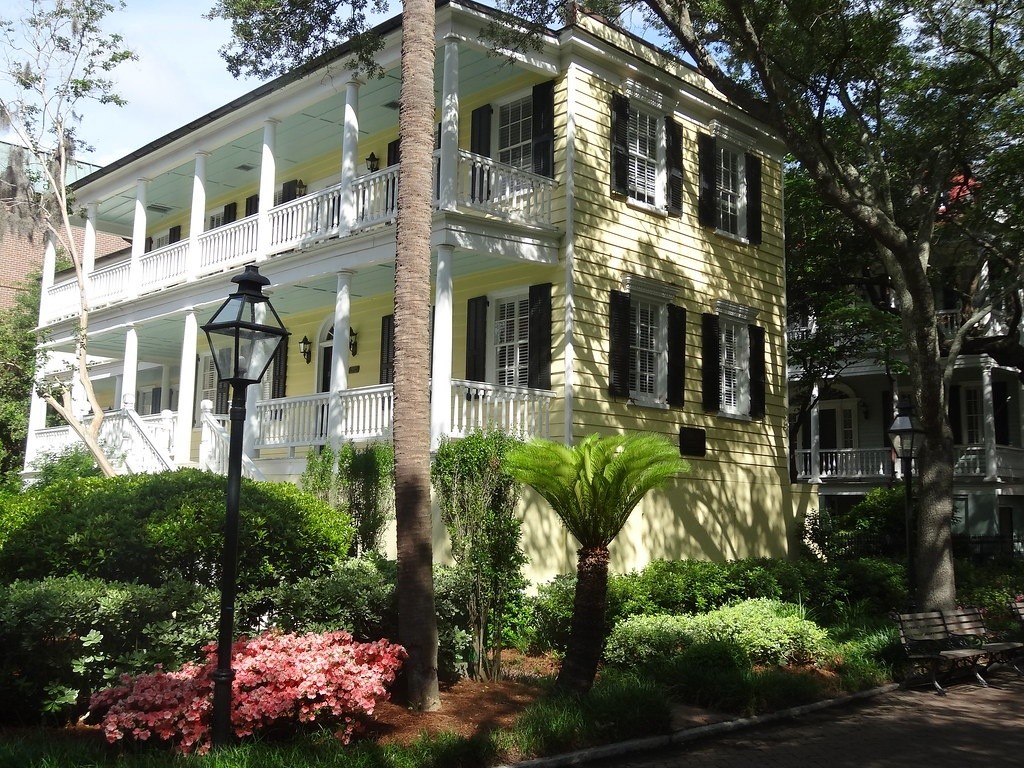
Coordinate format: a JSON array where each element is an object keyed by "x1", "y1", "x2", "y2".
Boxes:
[
  {"x1": 201, "y1": 264, "x2": 292, "y2": 752},
  {"x1": 887, "y1": 415, "x2": 929, "y2": 615}
]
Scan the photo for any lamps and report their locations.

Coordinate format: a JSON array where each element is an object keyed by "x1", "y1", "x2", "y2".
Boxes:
[
  {"x1": 366, "y1": 152, "x2": 379, "y2": 170},
  {"x1": 299, "y1": 335, "x2": 313, "y2": 365},
  {"x1": 297, "y1": 179, "x2": 307, "y2": 196},
  {"x1": 350, "y1": 326, "x2": 357, "y2": 357}
]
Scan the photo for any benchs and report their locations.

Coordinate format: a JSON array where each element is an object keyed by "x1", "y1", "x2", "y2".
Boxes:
[
  {"x1": 1011, "y1": 602, "x2": 1024, "y2": 623},
  {"x1": 889, "y1": 606, "x2": 1024, "y2": 695}
]
